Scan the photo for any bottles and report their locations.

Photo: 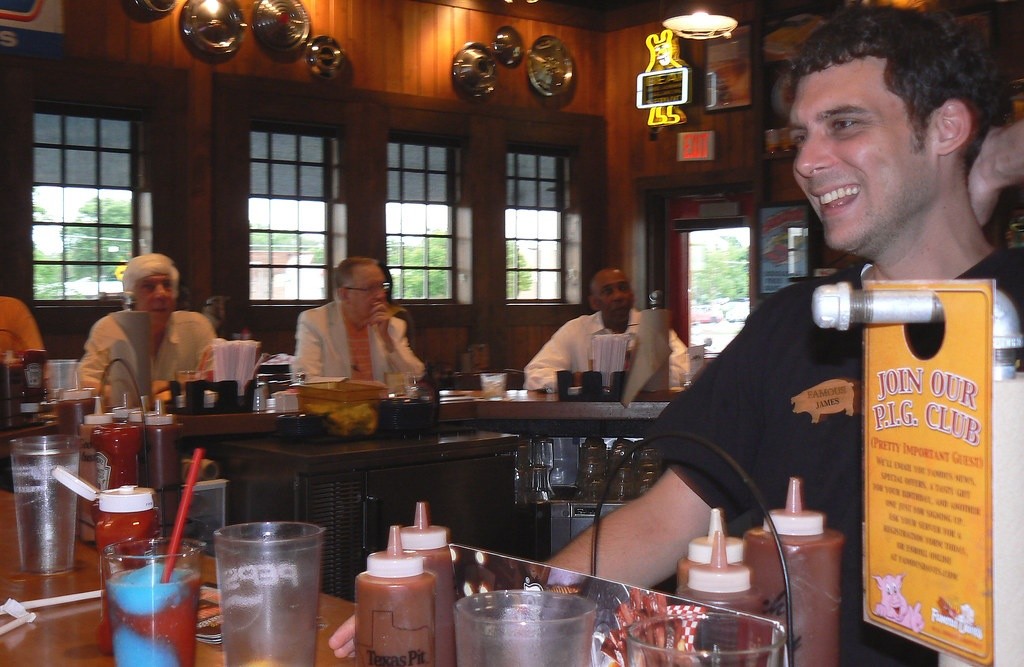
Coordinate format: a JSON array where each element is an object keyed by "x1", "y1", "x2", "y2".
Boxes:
[
  {"x1": 91, "y1": 424, "x2": 141, "y2": 490},
  {"x1": 677, "y1": 508, "x2": 744, "y2": 586},
  {"x1": 145, "y1": 400, "x2": 178, "y2": 489},
  {"x1": 295, "y1": 373, "x2": 305, "y2": 385},
  {"x1": 81, "y1": 397, "x2": 113, "y2": 487},
  {"x1": 400, "y1": 502, "x2": 456, "y2": 666},
  {"x1": 355, "y1": 525, "x2": 434, "y2": 667},
  {"x1": 53, "y1": 467, "x2": 160, "y2": 657},
  {"x1": 744, "y1": 478, "x2": 844, "y2": 667},
  {"x1": 128, "y1": 396, "x2": 156, "y2": 425},
  {"x1": 113, "y1": 393, "x2": 140, "y2": 424},
  {"x1": 253, "y1": 383, "x2": 265, "y2": 411},
  {"x1": 677, "y1": 531, "x2": 762, "y2": 616}
]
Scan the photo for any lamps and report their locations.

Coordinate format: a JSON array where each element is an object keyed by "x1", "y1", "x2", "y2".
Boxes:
[{"x1": 662, "y1": 0, "x2": 738, "y2": 39}]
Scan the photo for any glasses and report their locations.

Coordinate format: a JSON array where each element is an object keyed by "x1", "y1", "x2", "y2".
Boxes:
[{"x1": 342, "y1": 283, "x2": 390, "y2": 293}]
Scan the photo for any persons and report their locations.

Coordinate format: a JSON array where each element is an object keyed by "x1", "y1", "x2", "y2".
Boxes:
[
  {"x1": 328, "y1": 6, "x2": 1024, "y2": 667},
  {"x1": 77, "y1": 253, "x2": 219, "y2": 406},
  {"x1": 291, "y1": 256, "x2": 424, "y2": 381},
  {"x1": 0, "y1": 296, "x2": 48, "y2": 370},
  {"x1": 524, "y1": 268, "x2": 691, "y2": 391}
]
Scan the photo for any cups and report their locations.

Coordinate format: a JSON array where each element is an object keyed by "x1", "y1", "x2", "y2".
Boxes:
[
  {"x1": 402, "y1": 372, "x2": 424, "y2": 398},
  {"x1": 515, "y1": 438, "x2": 656, "y2": 502},
  {"x1": 453, "y1": 590, "x2": 598, "y2": 667},
  {"x1": 627, "y1": 612, "x2": 787, "y2": 667},
  {"x1": 176, "y1": 370, "x2": 205, "y2": 396},
  {"x1": 214, "y1": 521, "x2": 326, "y2": 667},
  {"x1": 480, "y1": 372, "x2": 508, "y2": 400},
  {"x1": 10, "y1": 435, "x2": 80, "y2": 575},
  {"x1": 101, "y1": 538, "x2": 207, "y2": 667}
]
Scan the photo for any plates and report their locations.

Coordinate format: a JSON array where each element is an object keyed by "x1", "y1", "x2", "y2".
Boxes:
[
  {"x1": 277, "y1": 414, "x2": 324, "y2": 437},
  {"x1": 381, "y1": 399, "x2": 433, "y2": 430}
]
